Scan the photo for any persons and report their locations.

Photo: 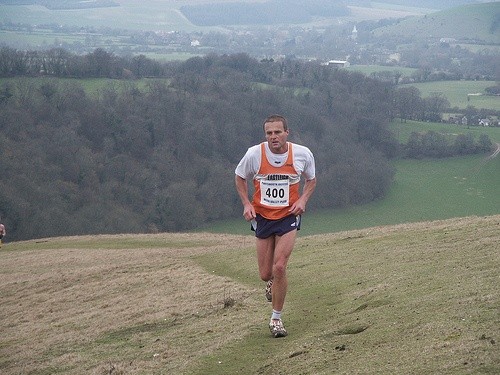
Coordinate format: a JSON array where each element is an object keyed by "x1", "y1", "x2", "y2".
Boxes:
[{"x1": 234, "y1": 115, "x2": 316, "y2": 337}]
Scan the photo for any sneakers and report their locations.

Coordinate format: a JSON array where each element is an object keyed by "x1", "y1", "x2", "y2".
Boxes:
[
  {"x1": 265, "y1": 280, "x2": 273, "y2": 302},
  {"x1": 269, "y1": 319, "x2": 288, "y2": 337}
]
